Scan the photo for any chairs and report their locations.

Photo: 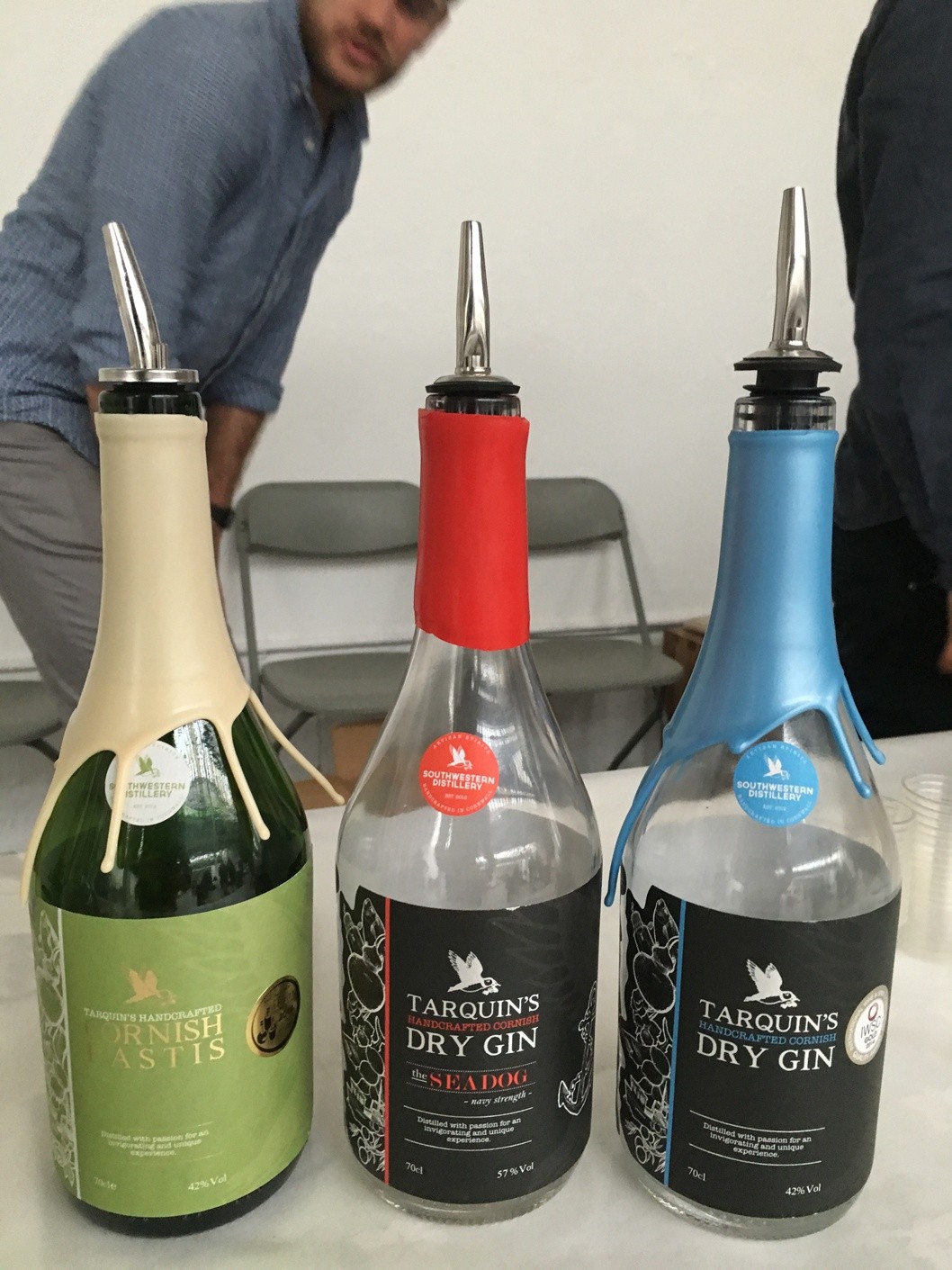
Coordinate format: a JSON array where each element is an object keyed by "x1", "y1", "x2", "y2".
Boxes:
[{"x1": 0, "y1": 476, "x2": 685, "y2": 809}]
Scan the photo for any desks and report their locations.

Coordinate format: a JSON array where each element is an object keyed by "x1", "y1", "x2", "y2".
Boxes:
[{"x1": 0, "y1": 728, "x2": 952, "y2": 1270}]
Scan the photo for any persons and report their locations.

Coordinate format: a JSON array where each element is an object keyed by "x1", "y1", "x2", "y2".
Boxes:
[
  {"x1": 0, "y1": 0, "x2": 462, "y2": 728},
  {"x1": 812, "y1": 0, "x2": 952, "y2": 742}
]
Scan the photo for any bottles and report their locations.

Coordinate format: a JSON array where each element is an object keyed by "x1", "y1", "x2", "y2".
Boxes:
[
  {"x1": 25, "y1": 220, "x2": 316, "y2": 1241},
  {"x1": 327, "y1": 220, "x2": 602, "y2": 1228},
  {"x1": 612, "y1": 190, "x2": 902, "y2": 1252}
]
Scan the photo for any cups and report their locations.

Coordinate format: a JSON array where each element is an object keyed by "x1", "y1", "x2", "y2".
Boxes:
[{"x1": 878, "y1": 767, "x2": 952, "y2": 965}]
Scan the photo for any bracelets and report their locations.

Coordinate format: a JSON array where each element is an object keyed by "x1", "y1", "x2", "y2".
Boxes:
[{"x1": 211, "y1": 502, "x2": 237, "y2": 529}]
